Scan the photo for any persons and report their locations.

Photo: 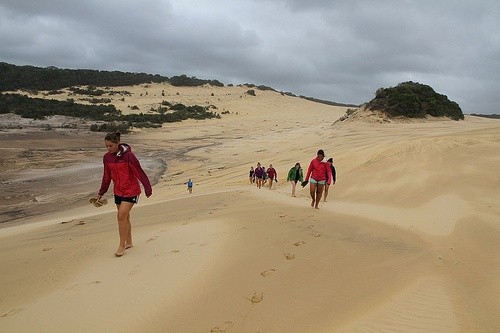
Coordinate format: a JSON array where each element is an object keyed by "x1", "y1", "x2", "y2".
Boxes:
[
  {"x1": 187, "y1": 179, "x2": 193, "y2": 194},
  {"x1": 323, "y1": 157, "x2": 336, "y2": 202},
  {"x1": 305, "y1": 150, "x2": 332, "y2": 209},
  {"x1": 96, "y1": 132, "x2": 152, "y2": 257},
  {"x1": 287, "y1": 162, "x2": 304, "y2": 197},
  {"x1": 249, "y1": 162, "x2": 277, "y2": 189}
]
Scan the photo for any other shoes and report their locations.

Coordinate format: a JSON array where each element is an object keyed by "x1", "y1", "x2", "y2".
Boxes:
[{"x1": 301, "y1": 181, "x2": 308, "y2": 188}]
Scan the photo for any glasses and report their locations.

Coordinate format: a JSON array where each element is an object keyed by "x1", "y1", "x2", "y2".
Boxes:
[{"x1": 107, "y1": 133, "x2": 118, "y2": 141}]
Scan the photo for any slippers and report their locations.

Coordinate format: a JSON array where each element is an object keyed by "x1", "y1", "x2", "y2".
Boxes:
[{"x1": 88, "y1": 197, "x2": 108, "y2": 207}]
